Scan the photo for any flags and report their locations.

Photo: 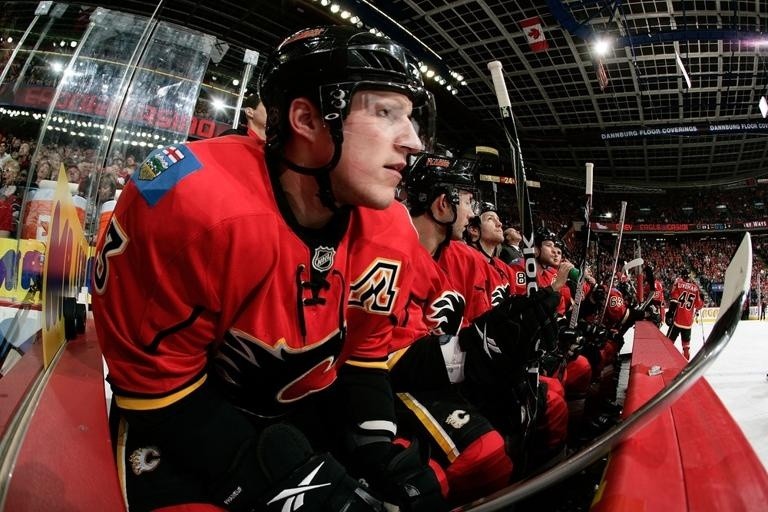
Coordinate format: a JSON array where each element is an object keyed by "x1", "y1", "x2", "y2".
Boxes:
[
  {"x1": 522, "y1": 15, "x2": 548, "y2": 52},
  {"x1": 589, "y1": 54, "x2": 609, "y2": 90}
]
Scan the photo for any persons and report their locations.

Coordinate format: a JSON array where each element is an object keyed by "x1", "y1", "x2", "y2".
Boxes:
[
  {"x1": 88, "y1": 26, "x2": 436, "y2": 509},
  {"x1": 466, "y1": 196, "x2": 572, "y2": 455},
  {"x1": 508, "y1": 227, "x2": 632, "y2": 331},
  {"x1": 1, "y1": 131, "x2": 140, "y2": 246},
  {"x1": 367, "y1": 146, "x2": 517, "y2": 448},
  {"x1": 643, "y1": 266, "x2": 665, "y2": 328},
  {"x1": 221, "y1": 91, "x2": 274, "y2": 144},
  {"x1": 665, "y1": 269, "x2": 703, "y2": 360},
  {"x1": 596, "y1": 186, "x2": 767, "y2": 307}
]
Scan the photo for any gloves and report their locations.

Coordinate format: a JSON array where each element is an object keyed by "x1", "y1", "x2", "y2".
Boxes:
[{"x1": 458, "y1": 284, "x2": 608, "y2": 382}]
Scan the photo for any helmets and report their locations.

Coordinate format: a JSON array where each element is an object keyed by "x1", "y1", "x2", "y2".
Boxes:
[
  {"x1": 466, "y1": 200, "x2": 497, "y2": 227},
  {"x1": 404, "y1": 141, "x2": 483, "y2": 216},
  {"x1": 260, "y1": 23, "x2": 438, "y2": 154},
  {"x1": 535, "y1": 226, "x2": 567, "y2": 252}
]
